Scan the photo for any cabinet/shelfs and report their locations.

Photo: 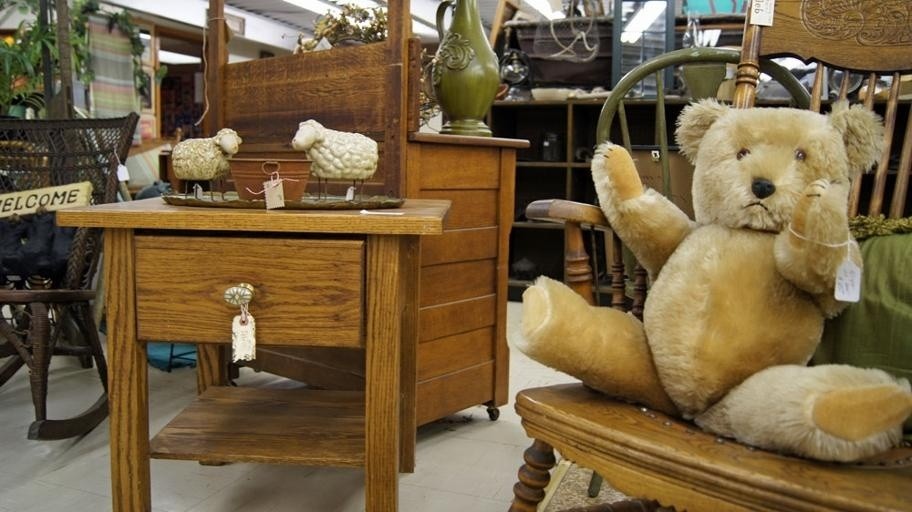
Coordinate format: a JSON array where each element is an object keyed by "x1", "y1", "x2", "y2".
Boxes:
[{"x1": 205, "y1": 0, "x2": 531, "y2": 430}]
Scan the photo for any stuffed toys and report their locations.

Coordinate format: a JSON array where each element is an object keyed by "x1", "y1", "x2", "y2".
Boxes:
[{"x1": 512, "y1": 99, "x2": 910, "y2": 462}]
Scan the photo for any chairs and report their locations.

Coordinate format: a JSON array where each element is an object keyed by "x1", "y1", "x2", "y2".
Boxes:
[{"x1": 0, "y1": 112, "x2": 139, "y2": 442}]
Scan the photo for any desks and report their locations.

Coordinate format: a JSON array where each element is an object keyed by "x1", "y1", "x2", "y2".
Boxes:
[{"x1": 53, "y1": 197, "x2": 452, "y2": 510}]
